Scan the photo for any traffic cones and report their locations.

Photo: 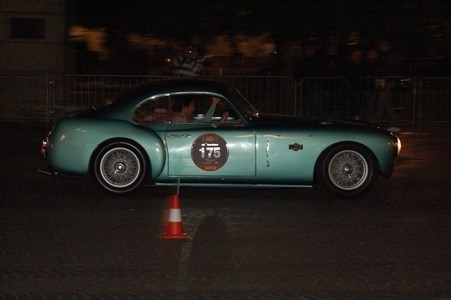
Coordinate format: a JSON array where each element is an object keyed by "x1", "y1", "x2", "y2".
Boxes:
[{"x1": 157, "y1": 193, "x2": 191, "y2": 240}]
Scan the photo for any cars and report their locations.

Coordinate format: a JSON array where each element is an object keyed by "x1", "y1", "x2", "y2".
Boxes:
[{"x1": 38, "y1": 77, "x2": 402, "y2": 201}]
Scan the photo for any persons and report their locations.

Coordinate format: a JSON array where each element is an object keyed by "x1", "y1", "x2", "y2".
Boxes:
[
  {"x1": 166, "y1": 44, "x2": 207, "y2": 78},
  {"x1": 361, "y1": 39, "x2": 399, "y2": 132},
  {"x1": 172, "y1": 97, "x2": 229, "y2": 126}
]
